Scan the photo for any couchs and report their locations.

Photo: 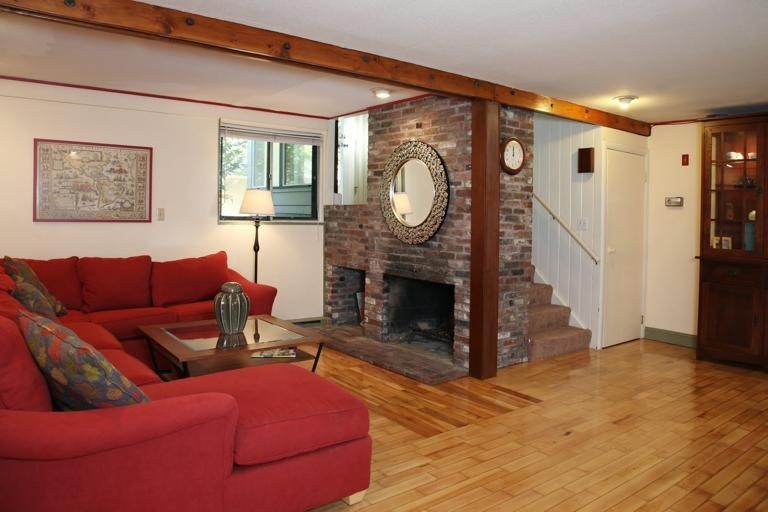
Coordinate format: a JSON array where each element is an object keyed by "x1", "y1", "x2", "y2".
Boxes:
[{"x1": 0, "y1": 268, "x2": 373, "y2": 511}]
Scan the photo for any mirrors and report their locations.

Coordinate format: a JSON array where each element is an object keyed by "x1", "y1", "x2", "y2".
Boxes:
[{"x1": 379, "y1": 140, "x2": 449, "y2": 246}]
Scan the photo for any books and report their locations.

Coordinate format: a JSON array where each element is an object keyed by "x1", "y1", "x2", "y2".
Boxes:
[{"x1": 250, "y1": 348, "x2": 297, "y2": 359}]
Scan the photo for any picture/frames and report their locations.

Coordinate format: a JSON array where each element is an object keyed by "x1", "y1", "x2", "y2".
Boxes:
[{"x1": 33, "y1": 137, "x2": 154, "y2": 223}]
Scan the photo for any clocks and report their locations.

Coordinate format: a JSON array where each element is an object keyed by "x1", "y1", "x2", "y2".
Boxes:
[{"x1": 500, "y1": 137, "x2": 526, "y2": 175}]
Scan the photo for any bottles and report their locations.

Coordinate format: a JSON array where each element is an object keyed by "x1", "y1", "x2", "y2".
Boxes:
[{"x1": 744, "y1": 223, "x2": 754, "y2": 251}]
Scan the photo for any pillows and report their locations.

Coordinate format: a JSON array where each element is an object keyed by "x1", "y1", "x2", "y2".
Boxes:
[{"x1": 0, "y1": 251, "x2": 228, "y2": 410}]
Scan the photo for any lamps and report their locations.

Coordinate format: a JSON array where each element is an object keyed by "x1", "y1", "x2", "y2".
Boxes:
[{"x1": 238, "y1": 189, "x2": 275, "y2": 282}]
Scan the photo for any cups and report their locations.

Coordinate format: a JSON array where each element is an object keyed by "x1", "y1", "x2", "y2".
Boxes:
[
  {"x1": 332, "y1": 193, "x2": 342, "y2": 205},
  {"x1": 353, "y1": 187, "x2": 361, "y2": 205}
]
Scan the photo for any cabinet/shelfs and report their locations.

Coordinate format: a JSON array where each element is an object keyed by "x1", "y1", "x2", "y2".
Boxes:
[{"x1": 697, "y1": 112, "x2": 768, "y2": 370}]
